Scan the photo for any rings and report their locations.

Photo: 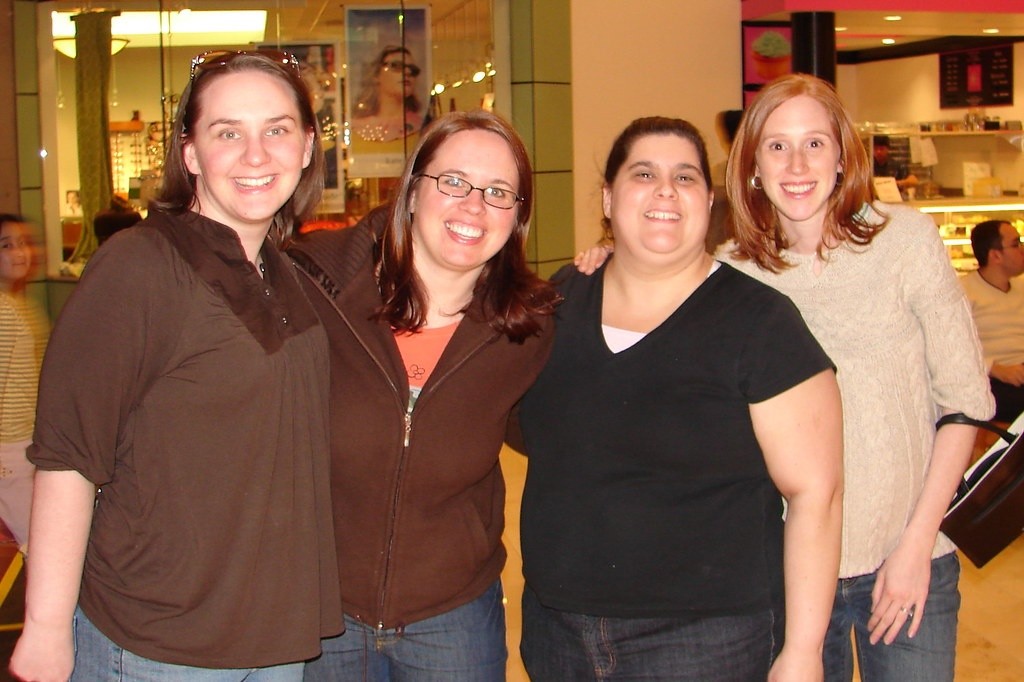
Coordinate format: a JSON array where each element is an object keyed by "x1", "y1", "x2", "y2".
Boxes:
[{"x1": 902, "y1": 607, "x2": 913, "y2": 616}]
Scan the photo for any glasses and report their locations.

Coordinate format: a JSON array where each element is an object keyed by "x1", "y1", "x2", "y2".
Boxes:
[
  {"x1": 382, "y1": 61, "x2": 420, "y2": 77},
  {"x1": 413, "y1": 173, "x2": 525, "y2": 210},
  {"x1": 190, "y1": 48, "x2": 301, "y2": 84}
]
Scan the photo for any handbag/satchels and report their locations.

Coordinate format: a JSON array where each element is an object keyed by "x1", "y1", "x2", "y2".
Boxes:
[{"x1": 935, "y1": 413, "x2": 1024, "y2": 568}]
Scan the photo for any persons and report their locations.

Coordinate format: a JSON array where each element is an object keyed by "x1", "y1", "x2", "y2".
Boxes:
[
  {"x1": 284, "y1": 109, "x2": 565, "y2": 682},
  {"x1": 0, "y1": 212, "x2": 55, "y2": 558},
  {"x1": 352, "y1": 47, "x2": 424, "y2": 143},
  {"x1": 94, "y1": 193, "x2": 142, "y2": 248},
  {"x1": 65, "y1": 190, "x2": 83, "y2": 217},
  {"x1": 957, "y1": 220, "x2": 1024, "y2": 423},
  {"x1": 575, "y1": 74, "x2": 997, "y2": 682},
  {"x1": 874, "y1": 134, "x2": 910, "y2": 194},
  {"x1": 9, "y1": 51, "x2": 345, "y2": 681},
  {"x1": 515, "y1": 117, "x2": 843, "y2": 682}
]
